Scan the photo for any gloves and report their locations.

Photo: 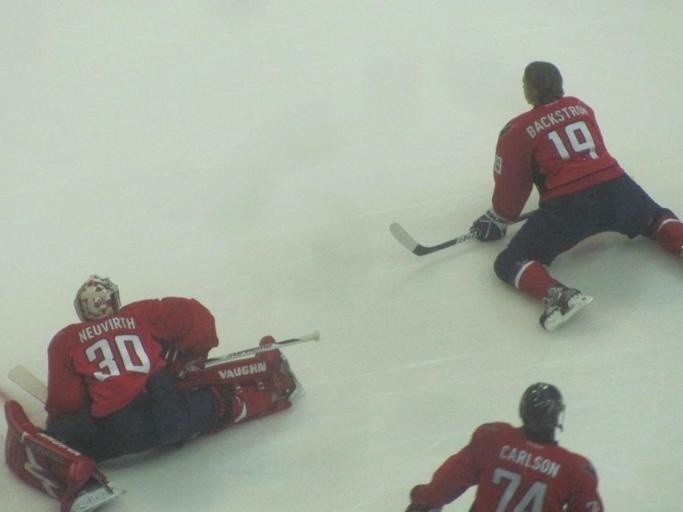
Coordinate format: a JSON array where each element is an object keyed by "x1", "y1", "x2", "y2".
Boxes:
[{"x1": 468, "y1": 208, "x2": 509, "y2": 242}]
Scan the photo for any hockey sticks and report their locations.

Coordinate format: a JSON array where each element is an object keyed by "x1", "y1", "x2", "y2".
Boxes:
[
  {"x1": 8, "y1": 332, "x2": 321, "y2": 405},
  {"x1": 388, "y1": 207, "x2": 534, "y2": 255}
]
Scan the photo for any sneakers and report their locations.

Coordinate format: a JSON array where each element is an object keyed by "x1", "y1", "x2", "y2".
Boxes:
[
  {"x1": 540, "y1": 283, "x2": 582, "y2": 325},
  {"x1": 74, "y1": 476, "x2": 104, "y2": 497}
]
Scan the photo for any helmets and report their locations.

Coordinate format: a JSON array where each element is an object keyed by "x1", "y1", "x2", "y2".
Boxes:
[
  {"x1": 519, "y1": 383, "x2": 564, "y2": 430},
  {"x1": 74, "y1": 276, "x2": 121, "y2": 323}
]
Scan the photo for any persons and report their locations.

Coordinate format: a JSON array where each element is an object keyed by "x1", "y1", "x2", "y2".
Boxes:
[
  {"x1": 466, "y1": 60, "x2": 681, "y2": 326},
  {"x1": 40, "y1": 274, "x2": 296, "y2": 497},
  {"x1": 400, "y1": 380, "x2": 603, "y2": 512}
]
